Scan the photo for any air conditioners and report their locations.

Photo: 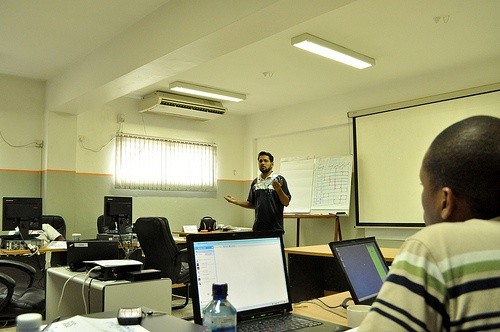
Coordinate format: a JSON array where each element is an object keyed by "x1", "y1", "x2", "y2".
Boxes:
[{"x1": 137, "y1": 91, "x2": 228, "y2": 124}]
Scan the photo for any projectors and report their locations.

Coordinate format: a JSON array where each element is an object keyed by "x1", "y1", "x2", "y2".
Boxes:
[{"x1": 82, "y1": 258, "x2": 144, "y2": 282}]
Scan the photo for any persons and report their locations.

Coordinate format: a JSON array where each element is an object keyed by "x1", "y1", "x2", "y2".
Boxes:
[
  {"x1": 357, "y1": 114, "x2": 500, "y2": 332},
  {"x1": 224, "y1": 151, "x2": 292, "y2": 277}
]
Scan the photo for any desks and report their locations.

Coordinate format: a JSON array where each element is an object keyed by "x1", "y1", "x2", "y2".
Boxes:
[
  {"x1": 0, "y1": 307, "x2": 208, "y2": 332},
  {"x1": 172, "y1": 227, "x2": 253, "y2": 246},
  {"x1": 285, "y1": 242, "x2": 400, "y2": 278},
  {"x1": 0, "y1": 244, "x2": 67, "y2": 257},
  {"x1": 44, "y1": 266, "x2": 172, "y2": 325}
]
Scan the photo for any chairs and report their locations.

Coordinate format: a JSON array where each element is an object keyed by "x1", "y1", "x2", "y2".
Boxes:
[
  {"x1": 134, "y1": 216, "x2": 190, "y2": 310},
  {"x1": 0, "y1": 260, "x2": 45, "y2": 317},
  {"x1": 42, "y1": 215, "x2": 66, "y2": 240}
]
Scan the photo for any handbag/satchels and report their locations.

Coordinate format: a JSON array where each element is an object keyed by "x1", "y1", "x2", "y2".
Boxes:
[{"x1": 200, "y1": 216, "x2": 217, "y2": 230}]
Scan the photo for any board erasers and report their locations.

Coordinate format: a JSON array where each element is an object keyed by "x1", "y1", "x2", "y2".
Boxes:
[{"x1": 336, "y1": 212, "x2": 346, "y2": 215}]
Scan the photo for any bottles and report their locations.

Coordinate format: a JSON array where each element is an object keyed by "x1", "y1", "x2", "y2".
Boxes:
[{"x1": 202, "y1": 283, "x2": 238, "y2": 332}]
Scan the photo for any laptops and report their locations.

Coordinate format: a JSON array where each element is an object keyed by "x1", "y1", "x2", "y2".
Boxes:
[
  {"x1": 329, "y1": 237, "x2": 389, "y2": 305},
  {"x1": 186, "y1": 229, "x2": 352, "y2": 332}
]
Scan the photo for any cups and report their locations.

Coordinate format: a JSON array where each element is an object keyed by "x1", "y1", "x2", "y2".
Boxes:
[
  {"x1": 217, "y1": 224, "x2": 224, "y2": 232},
  {"x1": 72, "y1": 233, "x2": 81, "y2": 241},
  {"x1": 346, "y1": 305, "x2": 372, "y2": 328}
]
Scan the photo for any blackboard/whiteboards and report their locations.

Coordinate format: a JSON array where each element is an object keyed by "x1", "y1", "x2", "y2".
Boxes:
[{"x1": 276, "y1": 156, "x2": 352, "y2": 217}]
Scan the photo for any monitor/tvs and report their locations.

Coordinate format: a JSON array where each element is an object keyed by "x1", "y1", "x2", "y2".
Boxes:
[
  {"x1": 103, "y1": 196, "x2": 132, "y2": 229},
  {"x1": 0, "y1": 197, "x2": 42, "y2": 239}
]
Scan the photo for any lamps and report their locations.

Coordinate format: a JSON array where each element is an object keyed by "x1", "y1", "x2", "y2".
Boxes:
[
  {"x1": 168, "y1": 81, "x2": 246, "y2": 104},
  {"x1": 292, "y1": 34, "x2": 376, "y2": 71}
]
